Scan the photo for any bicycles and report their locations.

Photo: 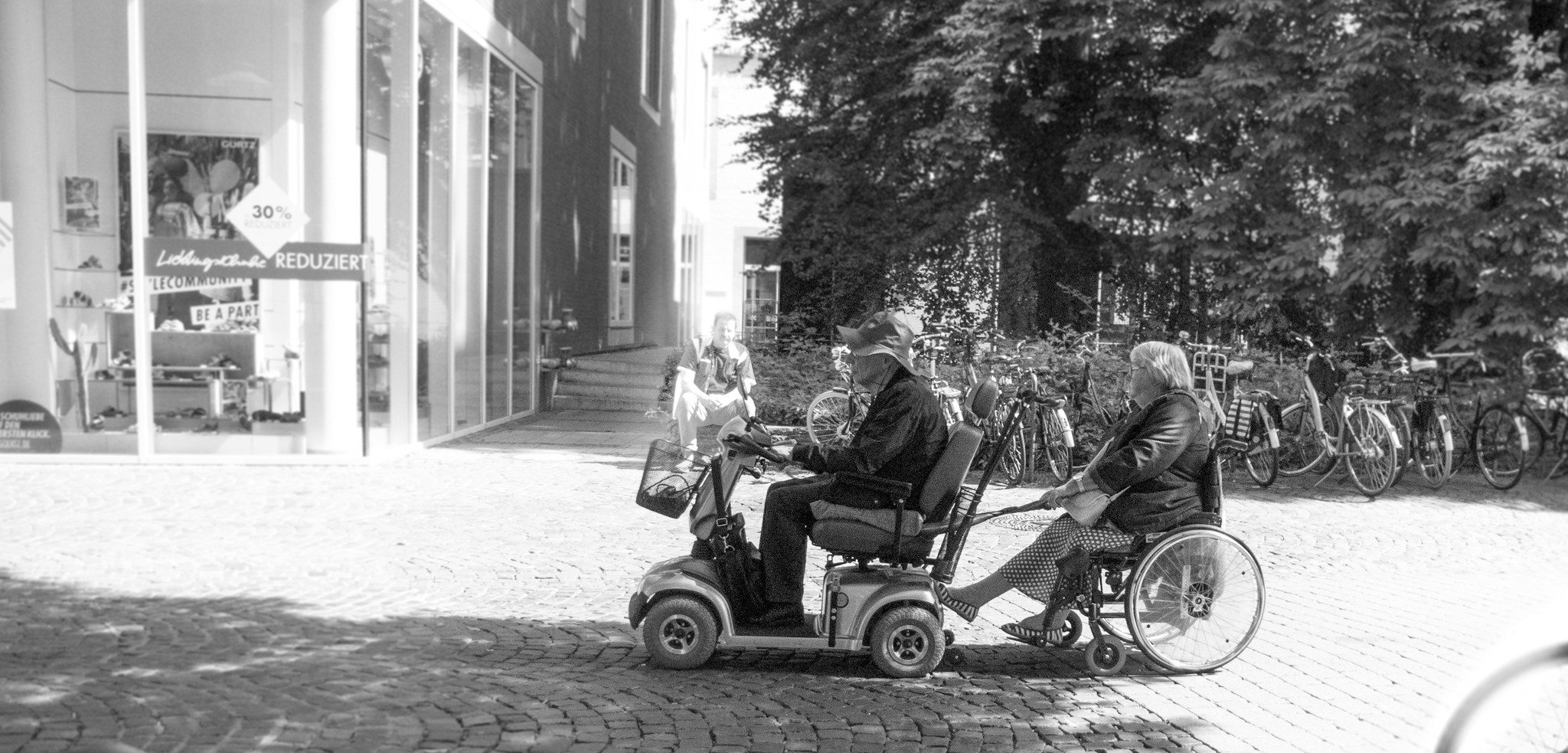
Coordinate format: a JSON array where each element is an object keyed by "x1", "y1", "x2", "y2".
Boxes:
[{"x1": 807, "y1": 329, "x2": 1568, "y2": 498}]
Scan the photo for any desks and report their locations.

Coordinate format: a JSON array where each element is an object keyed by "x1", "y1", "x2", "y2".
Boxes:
[{"x1": 108, "y1": 364, "x2": 241, "y2": 417}]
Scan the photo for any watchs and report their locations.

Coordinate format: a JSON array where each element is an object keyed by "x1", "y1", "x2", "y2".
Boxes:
[{"x1": 1073, "y1": 475, "x2": 1086, "y2": 494}]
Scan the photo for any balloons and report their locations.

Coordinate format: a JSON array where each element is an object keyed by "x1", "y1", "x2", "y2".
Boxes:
[{"x1": 148, "y1": 152, "x2": 245, "y2": 217}]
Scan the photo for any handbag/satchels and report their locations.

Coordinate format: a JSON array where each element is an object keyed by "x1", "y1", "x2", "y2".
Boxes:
[
  {"x1": 673, "y1": 337, "x2": 706, "y2": 419},
  {"x1": 713, "y1": 453, "x2": 766, "y2": 622},
  {"x1": 1056, "y1": 470, "x2": 1109, "y2": 528}
]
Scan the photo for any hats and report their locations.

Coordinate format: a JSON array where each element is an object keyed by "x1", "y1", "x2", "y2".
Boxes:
[{"x1": 836, "y1": 311, "x2": 921, "y2": 377}]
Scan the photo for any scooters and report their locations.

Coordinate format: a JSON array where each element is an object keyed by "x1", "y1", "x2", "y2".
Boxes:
[{"x1": 629, "y1": 374, "x2": 1069, "y2": 679}]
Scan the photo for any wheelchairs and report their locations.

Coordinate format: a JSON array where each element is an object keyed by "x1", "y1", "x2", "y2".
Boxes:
[{"x1": 1006, "y1": 438, "x2": 1266, "y2": 677}]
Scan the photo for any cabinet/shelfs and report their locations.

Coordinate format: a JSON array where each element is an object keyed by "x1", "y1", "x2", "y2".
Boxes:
[{"x1": 54, "y1": 227, "x2": 116, "y2": 308}]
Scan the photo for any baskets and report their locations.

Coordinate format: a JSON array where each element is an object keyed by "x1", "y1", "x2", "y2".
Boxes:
[
  {"x1": 982, "y1": 357, "x2": 1033, "y2": 392},
  {"x1": 636, "y1": 438, "x2": 712, "y2": 519}
]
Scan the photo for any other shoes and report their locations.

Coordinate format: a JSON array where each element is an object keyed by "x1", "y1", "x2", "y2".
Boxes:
[
  {"x1": 1002, "y1": 623, "x2": 1063, "y2": 646},
  {"x1": 675, "y1": 456, "x2": 694, "y2": 472},
  {"x1": 932, "y1": 579, "x2": 978, "y2": 623},
  {"x1": 748, "y1": 605, "x2": 804, "y2": 628}
]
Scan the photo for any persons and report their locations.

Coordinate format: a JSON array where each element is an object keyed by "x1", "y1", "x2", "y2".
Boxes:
[
  {"x1": 671, "y1": 312, "x2": 756, "y2": 471},
  {"x1": 935, "y1": 341, "x2": 1213, "y2": 646},
  {"x1": 744, "y1": 311, "x2": 950, "y2": 627},
  {"x1": 153, "y1": 179, "x2": 202, "y2": 241}
]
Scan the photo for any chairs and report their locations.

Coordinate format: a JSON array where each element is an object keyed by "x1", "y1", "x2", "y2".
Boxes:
[{"x1": 813, "y1": 378, "x2": 999, "y2": 560}]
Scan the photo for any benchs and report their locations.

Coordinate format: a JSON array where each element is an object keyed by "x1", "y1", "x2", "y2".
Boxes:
[{"x1": 670, "y1": 424, "x2": 826, "y2": 447}]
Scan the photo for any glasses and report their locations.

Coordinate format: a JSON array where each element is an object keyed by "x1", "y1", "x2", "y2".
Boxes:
[{"x1": 1125, "y1": 364, "x2": 1144, "y2": 375}]
